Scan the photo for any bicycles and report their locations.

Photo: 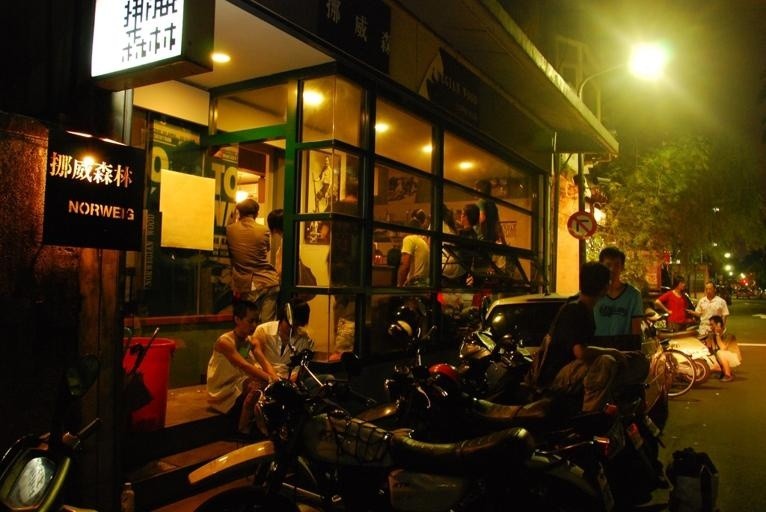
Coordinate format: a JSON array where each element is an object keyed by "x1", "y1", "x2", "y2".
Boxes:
[{"x1": 649, "y1": 312, "x2": 696, "y2": 396}]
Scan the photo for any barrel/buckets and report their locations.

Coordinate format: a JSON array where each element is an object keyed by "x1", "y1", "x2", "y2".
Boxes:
[{"x1": 123, "y1": 336, "x2": 175, "y2": 434}]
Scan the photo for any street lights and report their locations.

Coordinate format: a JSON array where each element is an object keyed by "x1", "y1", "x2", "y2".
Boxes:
[{"x1": 578, "y1": 39, "x2": 667, "y2": 290}]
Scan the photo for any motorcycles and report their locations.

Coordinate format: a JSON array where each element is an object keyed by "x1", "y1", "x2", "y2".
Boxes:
[
  {"x1": 1, "y1": 417, "x2": 104, "y2": 511},
  {"x1": 658, "y1": 329, "x2": 710, "y2": 386}
]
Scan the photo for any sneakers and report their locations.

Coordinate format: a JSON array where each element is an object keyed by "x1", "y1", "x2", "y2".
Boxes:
[{"x1": 719, "y1": 374, "x2": 736, "y2": 382}]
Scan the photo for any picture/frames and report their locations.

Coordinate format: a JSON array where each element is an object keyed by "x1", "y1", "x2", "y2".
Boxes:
[{"x1": 339, "y1": 152, "x2": 379, "y2": 206}]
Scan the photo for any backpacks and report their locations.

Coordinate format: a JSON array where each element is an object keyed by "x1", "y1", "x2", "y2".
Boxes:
[{"x1": 665, "y1": 446, "x2": 720, "y2": 512}]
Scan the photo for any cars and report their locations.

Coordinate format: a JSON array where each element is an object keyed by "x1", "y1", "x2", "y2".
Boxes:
[{"x1": 464, "y1": 293, "x2": 668, "y2": 422}]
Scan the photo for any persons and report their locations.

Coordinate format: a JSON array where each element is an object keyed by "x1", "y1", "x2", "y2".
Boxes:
[
  {"x1": 253, "y1": 298, "x2": 316, "y2": 384},
  {"x1": 528, "y1": 262, "x2": 611, "y2": 390},
  {"x1": 471, "y1": 178, "x2": 507, "y2": 289},
  {"x1": 593, "y1": 245, "x2": 647, "y2": 352},
  {"x1": 398, "y1": 202, "x2": 480, "y2": 287},
  {"x1": 204, "y1": 298, "x2": 281, "y2": 445},
  {"x1": 686, "y1": 281, "x2": 731, "y2": 337},
  {"x1": 255, "y1": 209, "x2": 283, "y2": 321},
  {"x1": 653, "y1": 274, "x2": 696, "y2": 334},
  {"x1": 225, "y1": 199, "x2": 281, "y2": 323},
  {"x1": 311, "y1": 154, "x2": 333, "y2": 214},
  {"x1": 704, "y1": 316, "x2": 743, "y2": 382}
]
